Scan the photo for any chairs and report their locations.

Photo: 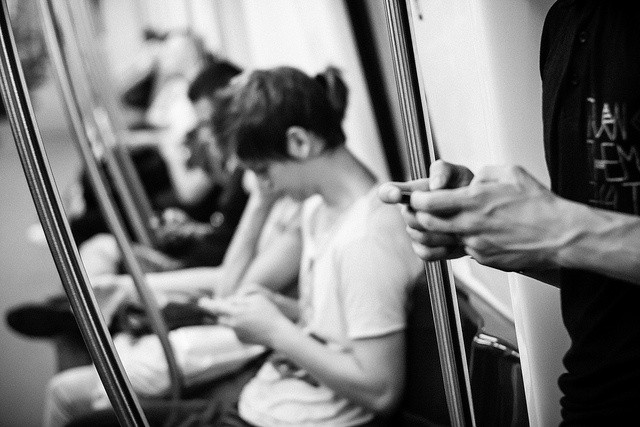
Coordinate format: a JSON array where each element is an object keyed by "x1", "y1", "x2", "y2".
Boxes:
[{"x1": 405, "y1": 279, "x2": 483, "y2": 427}]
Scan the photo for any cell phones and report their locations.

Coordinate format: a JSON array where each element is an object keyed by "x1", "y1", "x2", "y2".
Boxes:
[{"x1": 397, "y1": 191, "x2": 412, "y2": 206}]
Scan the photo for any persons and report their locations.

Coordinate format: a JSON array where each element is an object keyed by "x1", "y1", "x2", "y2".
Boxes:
[
  {"x1": 59, "y1": 68, "x2": 430, "y2": 425},
  {"x1": 30, "y1": 84, "x2": 316, "y2": 427},
  {"x1": 110, "y1": 30, "x2": 224, "y2": 148},
  {"x1": 379, "y1": 0, "x2": 640, "y2": 423},
  {"x1": 1, "y1": 62, "x2": 281, "y2": 376},
  {"x1": 126, "y1": 26, "x2": 168, "y2": 118}
]
